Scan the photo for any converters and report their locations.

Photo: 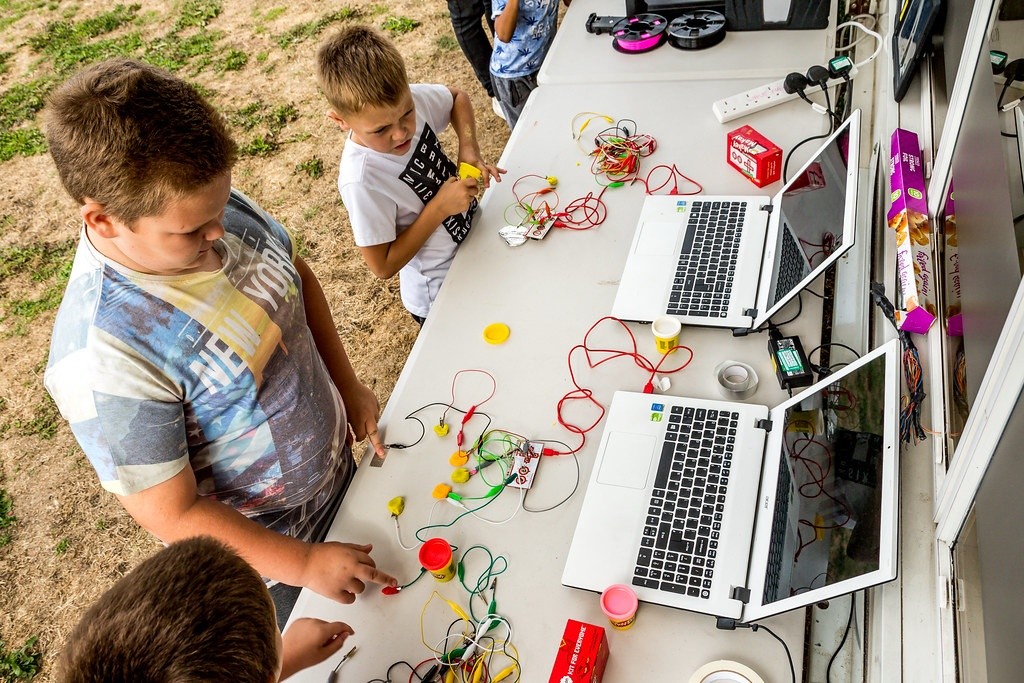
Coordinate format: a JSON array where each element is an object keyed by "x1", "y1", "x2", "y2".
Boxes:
[{"x1": 767, "y1": 335, "x2": 814, "y2": 391}]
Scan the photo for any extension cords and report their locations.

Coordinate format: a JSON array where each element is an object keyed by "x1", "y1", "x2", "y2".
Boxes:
[
  {"x1": 989, "y1": 53, "x2": 1024, "y2": 90},
  {"x1": 712, "y1": 58, "x2": 858, "y2": 123}
]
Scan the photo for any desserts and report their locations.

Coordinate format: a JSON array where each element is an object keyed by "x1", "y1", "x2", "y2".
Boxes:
[{"x1": 891, "y1": 210, "x2": 929, "y2": 246}]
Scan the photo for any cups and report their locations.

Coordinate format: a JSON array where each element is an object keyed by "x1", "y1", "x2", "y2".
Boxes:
[
  {"x1": 651, "y1": 316, "x2": 682, "y2": 354},
  {"x1": 419, "y1": 538, "x2": 456, "y2": 582},
  {"x1": 600, "y1": 584, "x2": 638, "y2": 631},
  {"x1": 788, "y1": 408, "x2": 825, "y2": 435}
]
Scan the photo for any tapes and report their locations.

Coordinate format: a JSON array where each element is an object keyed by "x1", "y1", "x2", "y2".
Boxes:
[
  {"x1": 722, "y1": 364, "x2": 748, "y2": 390},
  {"x1": 689, "y1": 660, "x2": 765, "y2": 683}
]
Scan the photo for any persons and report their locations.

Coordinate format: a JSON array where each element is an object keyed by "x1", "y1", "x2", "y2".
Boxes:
[
  {"x1": 489, "y1": 0, "x2": 572, "y2": 132},
  {"x1": 52, "y1": 534, "x2": 355, "y2": 683},
  {"x1": 446, "y1": 0, "x2": 506, "y2": 120},
  {"x1": 43, "y1": 60, "x2": 399, "y2": 633},
  {"x1": 316, "y1": 25, "x2": 507, "y2": 329}
]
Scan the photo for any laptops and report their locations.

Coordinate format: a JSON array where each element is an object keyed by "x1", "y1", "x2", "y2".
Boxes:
[
  {"x1": 561, "y1": 339, "x2": 901, "y2": 625},
  {"x1": 612, "y1": 110, "x2": 862, "y2": 332}
]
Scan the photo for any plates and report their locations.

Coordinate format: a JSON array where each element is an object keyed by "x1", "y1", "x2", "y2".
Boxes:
[{"x1": 687, "y1": 660, "x2": 765, "y2": 683}]
